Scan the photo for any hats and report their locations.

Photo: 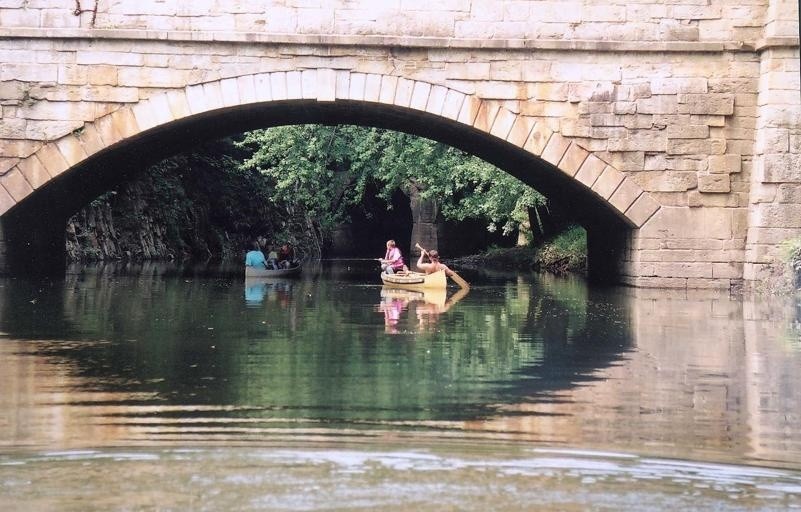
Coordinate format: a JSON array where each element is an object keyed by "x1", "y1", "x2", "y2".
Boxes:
[{"x1": 429, "y1": 250, "x2": 440, "y2": 259}]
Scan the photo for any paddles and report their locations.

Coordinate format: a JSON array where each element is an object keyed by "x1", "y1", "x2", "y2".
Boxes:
[{"x1": 415, "y1": 243, "x2": 470, "y2": 288}]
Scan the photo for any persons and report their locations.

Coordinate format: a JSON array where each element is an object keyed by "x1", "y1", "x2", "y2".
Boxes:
[
  {"x1": 416, "y1": 249, "x2": 455, "y2": 277},
  {"x1": 270, "y1": 244, "x2": 295, "y2": 270},
  {"x1": 383, "y1": 299, "x2": 405, "y2": 335},
  {"x1": 416, "y1": 301, "x2": 451, "y2": 332},
  {"x1": 243, "y1": 281, "x2": 268, "y2": 304},
  {"x1": 246, "y1": 243, "x2": 269, "y2": 270},
  {"x1": 381, "y1": 239, "x2": 405, "y2": 274},
  {"x1": 272, "y1": 281, "x2": 296, "y2": 310}
]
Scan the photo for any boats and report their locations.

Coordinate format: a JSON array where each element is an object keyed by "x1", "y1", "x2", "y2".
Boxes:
[
  {"x1": 242, "y1": 262, "x2": 307, "y2": 278},
  {"x1": 243, "y1": 278, "x2": 299, "y2": 301},
  {"x1": 377, "y1": 268, "x2": 448, "y2": 289},
  {"x1": 377, "y1": 287, "x2": 447, "y2": 312}
]
